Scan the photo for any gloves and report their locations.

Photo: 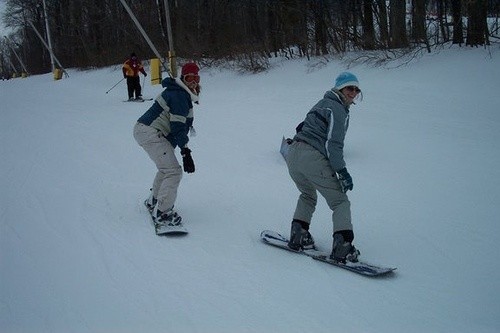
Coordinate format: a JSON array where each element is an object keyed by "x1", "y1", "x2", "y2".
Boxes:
[
  {"x1": 124, "y1": 73, "x2": 127, "y2": 78},
  {"x1": 143, "y1": 72, "x2": 147, "y2": 77},
  {"x1": 181, "y1": 148, "x2": 195, "y2": 173},
  {"x1": 337, "y1": 168, "x2": 353, "y2": 193}
]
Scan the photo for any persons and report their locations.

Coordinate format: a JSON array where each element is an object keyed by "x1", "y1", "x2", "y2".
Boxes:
[
  {"x1": 123, "y1": 54, "x2": 151, "y2": 99},
  {"x1": 133, "y1": 63, "x2": 201, "y2": 226},
  {"x1": 287, "y1": 72, "x2": 362, "y2": 262}
]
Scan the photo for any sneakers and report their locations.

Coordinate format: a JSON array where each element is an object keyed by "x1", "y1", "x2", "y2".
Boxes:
[
  {"x1": 146, "y1": 197, "x2": 157, "y2": 212},
  {"x1": 330, "y1": 234, "x2": 358, "y2": 262},
  {"x1": 152, "y1": 209, "x2": 182, "y2": 225},
  {"x1": 288, "y1": 222, "x2": 315, "y2": 250}
]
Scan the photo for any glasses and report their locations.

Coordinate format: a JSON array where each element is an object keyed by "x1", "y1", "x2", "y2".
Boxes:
[
  {"x1": 346, "y1": 86, "x2": 360, "y2": 93},
  {"x1": 184, "y1": 75, "x2": 200, "y2": 84}
]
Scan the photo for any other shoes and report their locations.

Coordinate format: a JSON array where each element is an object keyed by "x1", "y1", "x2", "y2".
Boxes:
[
  {"x1": 129, "y1": 97, "x2": 134, "y2": 100},
  {"x1": 136, "y1": 96, "x2": 142, "y2": 100}
]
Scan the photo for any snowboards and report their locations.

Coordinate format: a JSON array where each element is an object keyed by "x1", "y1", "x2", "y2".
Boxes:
[
  {"x1": 260, "y1": 229, "x2": 396, "y2": 277},
  {"x1": 144, "y1": 197, "x2": 189, "y2": 237}
]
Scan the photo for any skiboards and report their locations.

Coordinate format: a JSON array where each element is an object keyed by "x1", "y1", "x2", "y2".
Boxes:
[{"x1": 122, "y1": 97, "x2": 154, "y2": 103}]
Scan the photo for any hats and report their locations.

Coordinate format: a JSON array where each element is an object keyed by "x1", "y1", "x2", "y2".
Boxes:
[
  {"x1": 334, "y1": 71, "x2": 360, "y2": 90},
  {"x1": 131, "y1": 53, "x2": 136, "y2": 57},
  {"x1": 182, "y1": 63, "x2": 199, "y2": 75}
]
